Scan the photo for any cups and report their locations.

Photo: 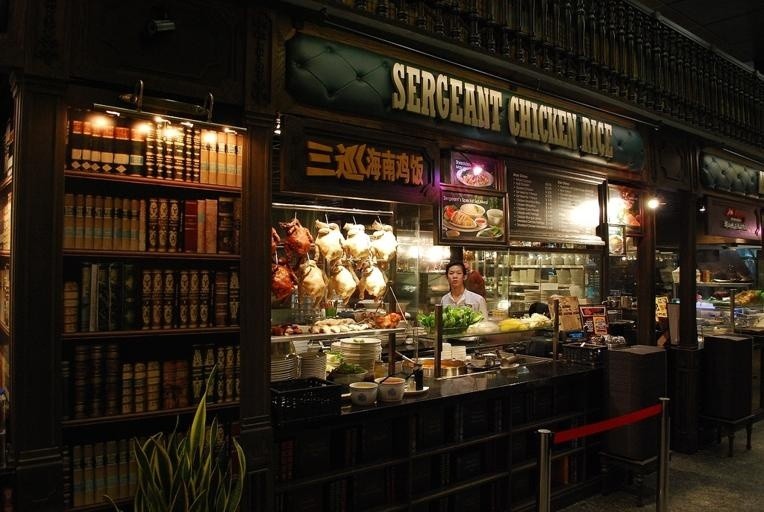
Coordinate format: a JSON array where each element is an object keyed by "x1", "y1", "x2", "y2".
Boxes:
[
  {"x1": 475, "y1": 218, "x2": 485, "y2": 226},
  {"x1": 445, "y1": 227, "x2": 460, "y2": 238},
  {"x1": 702, "y1": 269, "x2": 710, "y2": 282}
]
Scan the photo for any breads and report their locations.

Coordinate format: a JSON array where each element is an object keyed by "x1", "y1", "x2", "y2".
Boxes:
[{"x1": 450, "y1": 210, "x2": 477, "y2": 229}]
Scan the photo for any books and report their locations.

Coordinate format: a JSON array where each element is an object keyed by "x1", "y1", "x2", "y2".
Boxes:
[
  {"x1": 0, "y1": 190, "x2": 243, "y2": 254},
  {"x1": 62, "y1": 338, "x2": 244, "y2": 420},
  {"x1": 3, "y1": 111, "x2": 244, "y2": 188},
  {"x1": 0, "y1": 260, "x2": 11, "y2": 511},
  {"x1": 62, "y1": 258, "x2": 243, "y2": 334},
  {"x1": 63, "y1": 420, "x2": 245, "y2": 511}
]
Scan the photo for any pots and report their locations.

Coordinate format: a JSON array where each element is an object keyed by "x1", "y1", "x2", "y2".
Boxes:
[{"x1": 400, "y1": 358, "x2": 469, "y2": 378}]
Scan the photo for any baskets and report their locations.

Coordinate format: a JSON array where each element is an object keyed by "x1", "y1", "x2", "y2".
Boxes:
[{"x1": 561, "y1": 342, "x2": 606, "y2": 370}]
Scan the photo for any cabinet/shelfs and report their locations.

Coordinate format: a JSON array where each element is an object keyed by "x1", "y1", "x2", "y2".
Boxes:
[
  {"x1": 470, "y1": 250, "x2": 602, "y2": 324},
  {"x1": 607, "y1": 252, "x2": 679, "y2": 318},
  {"x1": 669, "y1": 280, "x2": 764, "y2": 336},
  {"x1": 396, "y1": 228, "x2": 450, "y2": 313}
]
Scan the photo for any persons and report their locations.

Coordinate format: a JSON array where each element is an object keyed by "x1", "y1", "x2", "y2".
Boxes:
[{"x1": 440, "y1": 262, "x2": 488, "y2": 322}]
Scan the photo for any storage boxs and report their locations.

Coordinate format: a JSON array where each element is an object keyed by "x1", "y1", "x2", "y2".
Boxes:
[{"x1": 13, "y1": 74, "x2": 268, "y2": 510}]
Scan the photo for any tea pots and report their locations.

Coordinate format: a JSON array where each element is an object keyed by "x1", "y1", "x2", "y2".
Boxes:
[{"x1": 471, "y1": 357, "x2": 496, "y2": 371}]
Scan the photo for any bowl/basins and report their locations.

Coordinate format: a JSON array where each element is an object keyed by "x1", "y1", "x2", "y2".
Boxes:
[
  {"x1": 485, "y1": 209, "x2": 503, "y2": 225},
  {"x1": 459, "y1": 203, "x2": 484, "y2": 218},
  {"x1": 349, "y1": 375, "x2": 406, "y2": 406},
  {"x1": 440, "y1": 342, "x2": 466, "y2": 363},
  {"x1": 470, "y1": 359, "x2": 488, "y2": 366}
]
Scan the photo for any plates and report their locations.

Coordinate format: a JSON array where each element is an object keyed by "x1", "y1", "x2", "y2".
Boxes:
[
  {"x1": 441, "y1": 219, "x2": 486, "y2": 232},
  {"x1": 510, "y1": 269, "x2": 583, "y2": 283},
  {"x1": 507, "y1": 254, "x2": 585, "y2": 266},
  {"x1": 475, "y1": 228, "x2": 504, "y2": 238},
  {"x1": 523, "y1": 289, "x2": 560, "y2": 310},
  {"x1": 455, "y1": 168, "x2": 494, "y2": 188},
  {"x1": 270, "y1": 338, "x2": 380, "y2": 386},
  {"x1": 405, "y1": 386, "x2": 430, "y2": 394},
  {"x1": 713, "y1": 278, "x2": 736, "y2": 283},
  {"x1": 357, "y1": 299, "x2": 383, "y2": 309}
]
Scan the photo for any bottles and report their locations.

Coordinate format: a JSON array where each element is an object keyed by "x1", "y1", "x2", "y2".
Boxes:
[
  {"x1": 325, "y1": 290, "x2": 337, "y2": 318},
  {"x1": 409, "y1": 365, "x2": 424, "y2": 390},
  {"x1": 695, "y1": 269, "x2": 701, "y2": 283}
]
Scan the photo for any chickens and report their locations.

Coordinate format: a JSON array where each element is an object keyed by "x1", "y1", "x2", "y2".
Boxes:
[
  {"x1": 279, "y1": 218, "x2": 314, "y2": 260},
  {"x1": 371, "y1": 220, "x2": 399, "y2": 272},
  {"x1": 327, "y1": 265, "x2": 357, "y2": 305},
  {"x1": 358, "y1": 266, "x2": 389, "y2": 303},
  {"x1": 271, "y1": 262, "x2": 298, "y2": 307},
  {"x1": 313, "y1": 219, "x2": 347, "y2": 265},
  {"x1": 342, "y1": 222, "x2": 371, "y2": 270},
  {"x1": 297, "y1": 259, "x2": 328, "y2": 309}
]
